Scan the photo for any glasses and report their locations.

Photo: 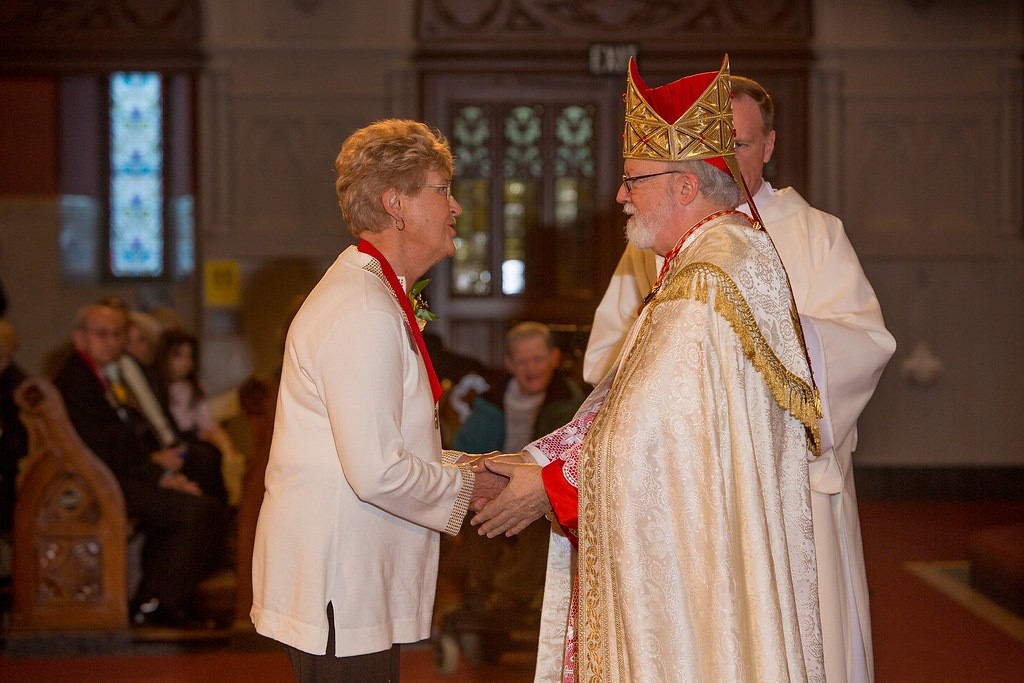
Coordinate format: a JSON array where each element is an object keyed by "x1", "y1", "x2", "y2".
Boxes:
[
  {"x1": 417, "y1": 181, "x2": 452, "y2": 200},
  {"x1": 622, "y1": 170, "x2": 683, "y2": 194}
]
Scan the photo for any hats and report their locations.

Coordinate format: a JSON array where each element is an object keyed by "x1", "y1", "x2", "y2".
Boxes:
[{"x1": 622, "y1": 54, "x2": 735, "y2": 161}]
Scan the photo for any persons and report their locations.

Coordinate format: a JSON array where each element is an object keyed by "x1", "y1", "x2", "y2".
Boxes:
[
  {"x1": 0, "y1": 281, "x2": 578, "y2": 635},
  {"x1": 247, "y1": 52, "x2": 898, "y2": 683}
]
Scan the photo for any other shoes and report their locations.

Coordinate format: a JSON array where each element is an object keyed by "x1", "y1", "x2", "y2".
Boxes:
[
  {"x1": 457, "y1": 578, "x2": 520, "y2": 614},
  {"x1": 129, "y1": 594, "x2": 210, "y2": 630}
]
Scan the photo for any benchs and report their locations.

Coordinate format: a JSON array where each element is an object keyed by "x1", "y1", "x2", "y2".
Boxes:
[{"x1": 11, "y1": 374, "x2": 146, "y2": 631}]
